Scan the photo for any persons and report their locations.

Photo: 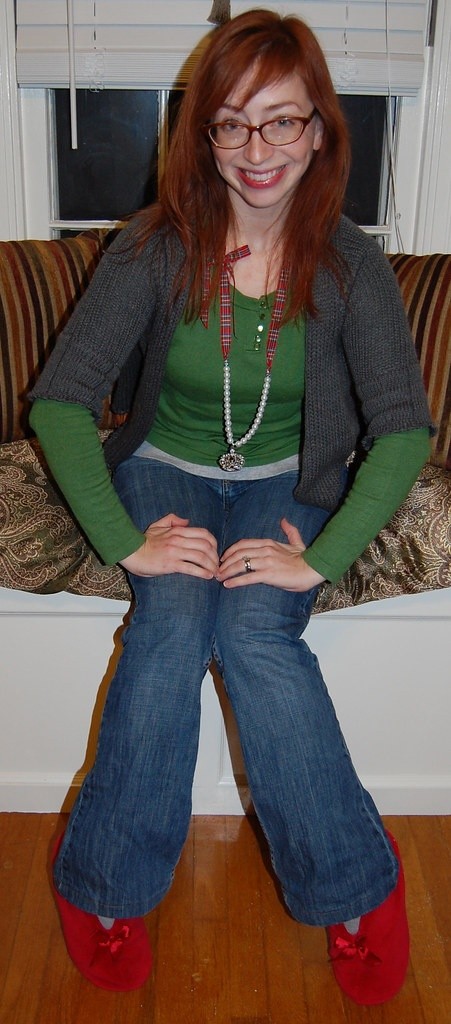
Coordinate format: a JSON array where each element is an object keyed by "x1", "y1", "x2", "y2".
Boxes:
[
  {"x1": 30, "y1": 11, "x2": 432, "y2": 1007},
  {"x1": 212, "y1": 228, "x2": 294, "y2": 471}
]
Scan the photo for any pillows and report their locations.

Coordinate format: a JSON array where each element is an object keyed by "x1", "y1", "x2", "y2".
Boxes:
[
  {"x1": 0, "y1": 229, "x2": 129, "y2": 447},
  {"x1": 385, "y1": 253, "x2": 451, "y2": 469}
]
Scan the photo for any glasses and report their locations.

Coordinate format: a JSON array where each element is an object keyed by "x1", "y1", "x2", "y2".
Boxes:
[{"x1": 202, "y1": 106, "x2": 318, "y2": 149}]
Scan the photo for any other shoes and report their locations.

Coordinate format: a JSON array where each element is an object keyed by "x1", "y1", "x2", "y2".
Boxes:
[
  {"x1": 48, "y1": 829, "x2": 152, "y2": 991},
  {"x1": 328, "y1": 827, "x2": 409, "y2": 1007}
]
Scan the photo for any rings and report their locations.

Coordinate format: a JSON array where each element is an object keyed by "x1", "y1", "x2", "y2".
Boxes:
[{"x1": 242, "y1": 556, "x2": 251, "y2": 573}]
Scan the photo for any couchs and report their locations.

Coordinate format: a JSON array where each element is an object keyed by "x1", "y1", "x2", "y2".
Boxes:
[{"x1": 1, "y1": 230, "x2": 450, "y2": 817}]
